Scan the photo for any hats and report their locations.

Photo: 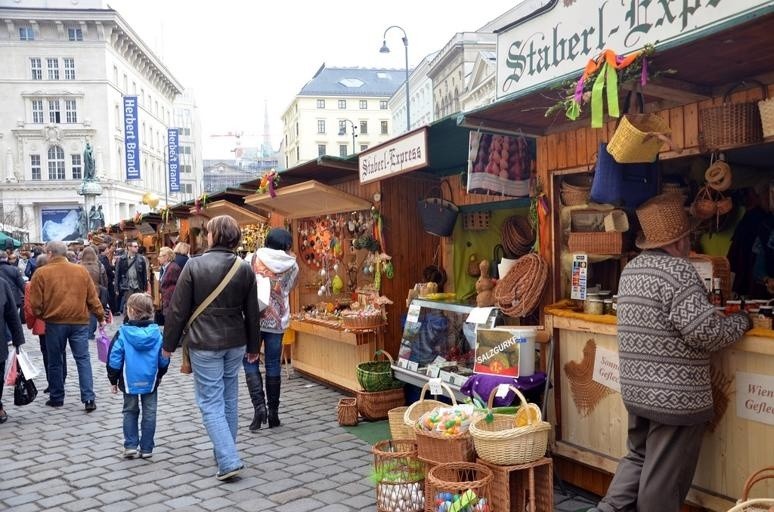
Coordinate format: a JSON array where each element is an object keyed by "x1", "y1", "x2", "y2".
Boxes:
[{"x1": 633, "y1": 194, "x2": 703, "y2": 251}]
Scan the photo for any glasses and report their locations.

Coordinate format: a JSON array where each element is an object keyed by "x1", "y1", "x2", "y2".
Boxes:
[{"x1": 130, "y1": 246, "x2": 138, "y2": 248}]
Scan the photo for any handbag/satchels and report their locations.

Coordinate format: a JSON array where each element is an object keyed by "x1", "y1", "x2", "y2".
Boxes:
[
  {"x1": 4, "y1": 346, "x2": 18, "y2": 387},
  {"x1": 417, "y1": 178, "x2": 460, "y2": 238},
  {"x1": 96, "y1": 326, "x2": 110, "y2": 362},
  {"x1": 250, "y1": 254, "x2": 271, "y2": 314},
  {"x1": 14, "y1": 346, "x2": 37, "y2": 406},
  {"x1": 17, "y1": 346, "x2": 41, "y2": 381},
  {"x1": 180, "y1": 256, "x2": 244, "y2": 374},
  {"x1": 99, "y1": 262, "x2": 111, "y2": 309}
]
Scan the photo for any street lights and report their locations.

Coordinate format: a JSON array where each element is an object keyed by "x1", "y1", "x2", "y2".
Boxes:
[{"x1": 380, "y1": 25, "x2": 410, "y2": 132}]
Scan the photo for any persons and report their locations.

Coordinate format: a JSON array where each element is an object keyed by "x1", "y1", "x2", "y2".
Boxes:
[
  {"x1": 9, "y1": 254, "x2": 17, "y2": 266},
  {"x1": 243, "y1": 229, "x2": 299, "y2": 429},
  {"x1": 174, "y1": 242, "x2": 190, "y2": 267},
  {"x1": 99, "y1": 246, "x2": 121, "y2": 316},
  {"x1": 30, "y1": 241, "x2": 106, "y2": 410},
  {"x1": 81, "y1": 246, "x2": 108, "y2": 340},
  {"x1": 138, "y1": 245, "x2": 150, "y2": 294},
  {"x1": 162, "y1": 215, "x2": 261, "y2": 480},
  {"x1": 5, "y1": 237, "x2": 13, "y2": 250},
  {"x1": 24, "y1": 246, "x2": 42, "y2": 281},
  {"x1": 77, "y1": 240, "x2": 84, "y2": 250},
  {"x1": 115, "y1": 241, "x2": 147, "y2": 322},
  {"x1": 84, "y1": 143, "x2": 95, "y2": 181},
  {"x1": 0, "y1": 252, "x2": 24, "y2": 344},
  {"x1": 107, "y1": 292, "x2": 170, "y2": 458},
  {"x1": 98, "y1": 205, "x2": 104, "y2": 228},
  {"x1": 589, "y1": 193, "x2": 758, "y2": 512},
  {"x1": 762, "y1": 271, "x2": 774, "y2": 295},
  {"x1": 67, "y1": 242, "x2": 78, "y2": 263},
  {"x1": 0, "y1": 278, "x2": 25, "y2": 423},
  {"x1": 158, "y1": 247, "x2": 183, "y2": 347},
  {"x1": 89, "y1": 204, "x2": 99, "y2": 229},
  {"x1": 25, "y1": 254, "x2": 67, "y2": 393}
]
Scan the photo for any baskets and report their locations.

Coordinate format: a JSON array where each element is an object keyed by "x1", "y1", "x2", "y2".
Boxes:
[
  {"x1": 418, "y1": 425, "x2": 473, "y2": 464},
  {"x1": 343, "y1": 312, "x2": 387, "y2": 330},
  {"x1": 687, "y1": 253, "x2": 731, "y2": 307},
  {"x1": 589, "y1": 141, "x2": 651, "y2": 207},
  {"x1": 403, "y1": 379, "x2": 460, "y2": 439},
  {"x1": 494, "y1": 255, "x2": 549, "y2": 316},
  {"x1": 726, "y1": 466, "x2": 774, "y2": 512},
  {"x1": 605, "y1": 79, "x2": 684, "y2": 165},
  {"x1": 338, "y1": 398, "x2": 358, "y2": 426},
  {"x1": 691, "y1": 185, "x2": 734, "y2": 217},
  {"x1": 357, "y1": 388, "x2": 405, "y2": 422},
  {"x1": 695, "y1": 80, "x2": 767, "y2": 151},
  {"x1": 604, "y1": 210, "x2": 629, "y2": 232},
  {"x1": 387, "y1": 405, "x2": 409, "y2": 439},
  {"x1": 505, "y1": 215, "x2": 537, "y2": 258},
  {"x1": 756, "y1": 95, "x2": 773, "y2": 138},
  {"x1": 357, "y1": 349, "x2": 402, "y2": 390},
  {"x1": 467, "y1": 253, "x2": 488, "y2": 276},
  {"x1": 560, "y1": 173, "x2": 590, "y2": 206},
  {"x1": 475, "y1": 458, "x2": 554, "y2": 512},
  {"x1": 469, "y1": 385, "x2": 551, "y2": 465},
  {"x1": 371, "y1": 439, "x2": 419, "y2": 512},
  {"x1": 568, "y1": 207, "x2": 624, "y2": 256}
]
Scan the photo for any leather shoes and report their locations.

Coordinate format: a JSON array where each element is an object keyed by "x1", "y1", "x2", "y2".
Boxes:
[
  {"x1": 85, "y1": 400, "x2": 97, "y2": 411},
  {"x1": 45, "y1": 399, "x2": 63, "y2": 406},
  {"x1": 1, "y1": 411, "x2": 8, "y2": 423}
]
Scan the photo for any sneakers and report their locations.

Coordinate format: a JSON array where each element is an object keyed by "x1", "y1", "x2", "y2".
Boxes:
[
  {"x1": 123, "y1": 449, "x2": 139, "y2": 458},
  {"x1": 216, "y1": 466, "x2": 245, "y2": 481},
  {"x1": 141, "y1": 453, "x2": 154, "y2": 459}
]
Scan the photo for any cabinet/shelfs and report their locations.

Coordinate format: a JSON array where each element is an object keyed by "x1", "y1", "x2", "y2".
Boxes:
[
  {"x1": 289, "y1": 312, "x2": 376, "y2": 396},
  {"x1": 538, "y1": 298, "x2": 774, "y2": 512}
]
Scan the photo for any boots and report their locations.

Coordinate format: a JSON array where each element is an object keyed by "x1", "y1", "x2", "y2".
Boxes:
[
  {"x1": 244, "y1": 372, "x2": 268, "y2": 430},
  {"x1": 265, "y1": 375, "x2": 281, "y2": 427}
]
